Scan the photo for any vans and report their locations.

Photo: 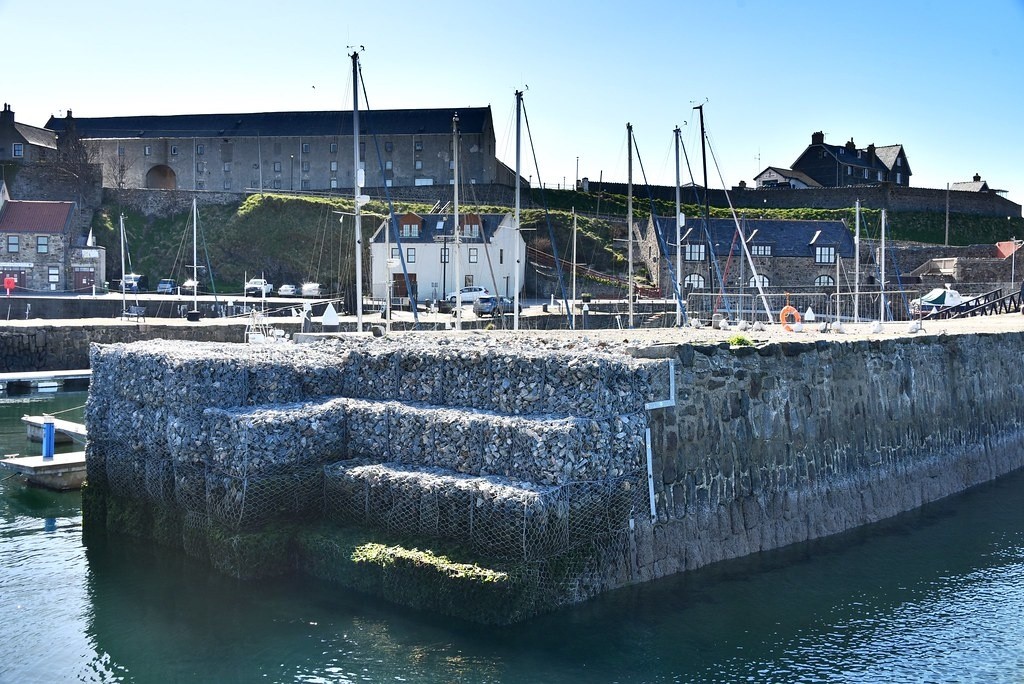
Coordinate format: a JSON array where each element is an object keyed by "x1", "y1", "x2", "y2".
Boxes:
[{"x1": 118, "y1": 273, "x2": 149, "y2": 294}]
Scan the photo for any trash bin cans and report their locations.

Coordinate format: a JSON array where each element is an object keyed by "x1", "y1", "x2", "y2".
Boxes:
[{"x1": 103, "y1": 282, "x2": 109, "y2": 293}]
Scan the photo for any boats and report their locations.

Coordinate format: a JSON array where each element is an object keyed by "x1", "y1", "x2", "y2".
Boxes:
[{"x1": 907, "y1": 287, "x2": 991, "y2": 322}]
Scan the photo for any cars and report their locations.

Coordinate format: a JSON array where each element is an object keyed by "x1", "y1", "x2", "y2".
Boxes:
[
  {"x1": 156, "y1": 278, "x2": 178, "y2": 295},
  {"x1": 446, "y1": 286, "x2": 490, "y2": 303},
  {"x1": 472, "y1": 294, "x2": 523, "y2": 318},
  {"x1": 277, "y1": 284, "x2": 299, "y2": 298},
  {"x1": 180, "y1": 280, "x2": 208, "y2": 295},
  {"x1": 301, "y1": 282, "x2": 329, "y2": 299}
]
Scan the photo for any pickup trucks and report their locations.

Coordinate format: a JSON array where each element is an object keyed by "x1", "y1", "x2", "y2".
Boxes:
[{"x1": 244, "y1": 278, "x2": 274, "y2": 297}]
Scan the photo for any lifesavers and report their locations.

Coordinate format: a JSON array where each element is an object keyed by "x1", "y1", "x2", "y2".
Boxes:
[{"x1": 779, "y1": 305, "x2": 801, "y2": 331}]
